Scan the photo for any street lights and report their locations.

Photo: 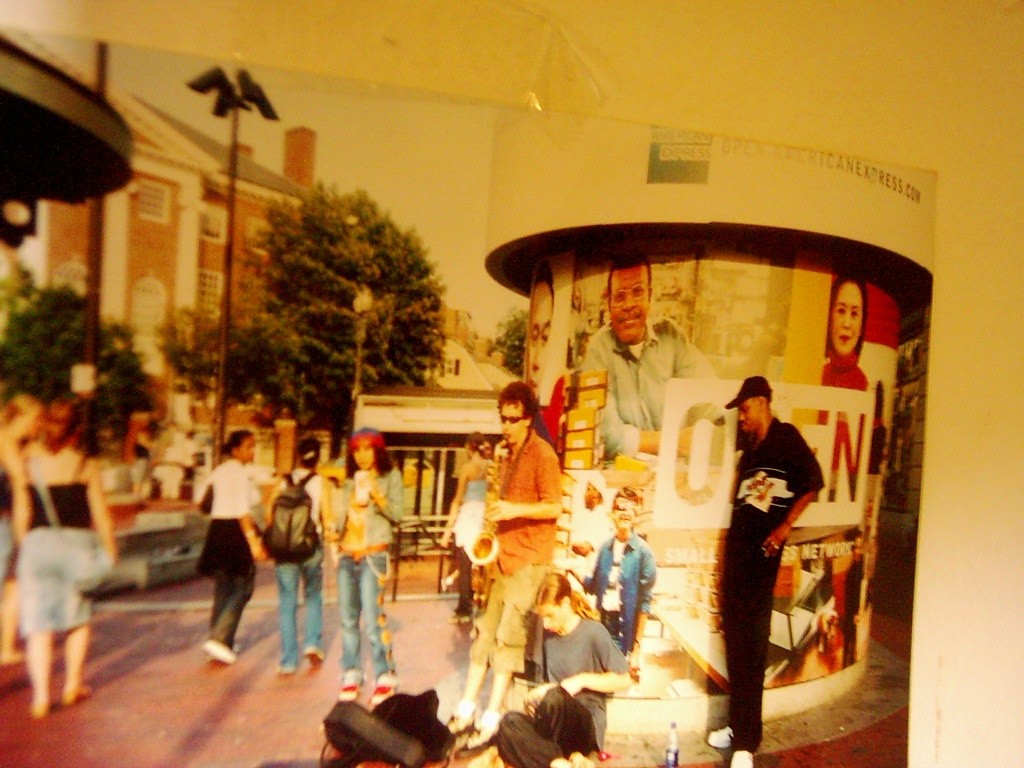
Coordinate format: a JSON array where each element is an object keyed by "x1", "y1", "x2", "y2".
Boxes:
[{"x1": 186, "y1": 64, "x2": 283, "y2": 470}]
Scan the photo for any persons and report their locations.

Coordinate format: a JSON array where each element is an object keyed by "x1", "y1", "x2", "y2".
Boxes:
[
  {"x1": 436, "y1": 431, "x2": 494, "y2": 623},
  {"x1": 522, "y1": 260, "x2": 556, "y2": 395},
  {"x1": 90, "y1": 323, "x2": 147, "y2": 395},
  {"x1": 708, "y1": 376, "x2": 826, "y2": 768},
  {"x1": 842, "y1": 527, "x2": 865, "y2": 668},
  {"x1": 803, "y1": 539, "x2": 833, "y2": 613},
  {"x1": 446, "y1": 381, "x2": 561, "y2": 750},
  {"x1": 582, "y1": 247, "x2": 708, "y2": 453},
  {"x1": 75, "y1": 386, "x2": 276, "y2": 498},
  {"x1": 334, "y1": 427, "x2": 404, "y2": 704},
  {"x1": 193, "y1": 428, "x2": 267, "y2": 664},
  {"x1": 823, "y1": 247, "x2": 872, "y2": 391},
  {"x1": 567, "y1": 486, "x2": 657, "y2": 660},
  {"x1": 495, "y1": 575, "x2": 633, "y2": 768},
  {"x1": 264, "y1": 436, "x2": 335, "y2": 676},
  {"x1": 0, "y1": 392, "x2": 120, "y2": 718}
]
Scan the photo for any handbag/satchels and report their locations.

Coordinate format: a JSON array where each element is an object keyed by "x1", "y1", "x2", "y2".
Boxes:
[{"x1": 57, "y1": 532, "x2": 112, "y2": 590}]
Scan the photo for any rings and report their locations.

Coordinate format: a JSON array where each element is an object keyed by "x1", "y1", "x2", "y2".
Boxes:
[
  {"x1": 774, "y1": 546, "x2": 780, "y2": 549},
  {"x1": 771, "y1": 542, "x2": 774, "y2": 544}
]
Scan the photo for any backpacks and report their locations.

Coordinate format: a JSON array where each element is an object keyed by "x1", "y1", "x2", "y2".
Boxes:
[{"x1": 262, "y1": 473, "x2": 320, "y2": 563}]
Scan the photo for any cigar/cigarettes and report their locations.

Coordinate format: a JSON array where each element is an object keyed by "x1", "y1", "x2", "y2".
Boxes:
[{"x1": 762, "y1": 547, "x2": 766, "y2": 551}]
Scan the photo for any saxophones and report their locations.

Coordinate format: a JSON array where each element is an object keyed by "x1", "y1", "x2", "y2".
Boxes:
[{"x1": 465, "y1": 437, "x2": 512, "y2": 606}]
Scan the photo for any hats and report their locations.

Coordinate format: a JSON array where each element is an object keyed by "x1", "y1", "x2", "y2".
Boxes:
[
  {"x1": 725, "y1": 376, "x2": 773, "y2": 410},
  {"x1": 347, "y1": 426, "x2": 385, "y2": 452}
]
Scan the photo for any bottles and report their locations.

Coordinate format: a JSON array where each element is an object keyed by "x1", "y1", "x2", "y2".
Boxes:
[{"x1": 665, "y1": 721, "x2": 680, "y2": 768}]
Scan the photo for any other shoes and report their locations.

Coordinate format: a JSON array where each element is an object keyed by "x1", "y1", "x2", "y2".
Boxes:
[
  {"x1": 0, "y1": 649, "x2": 25, "y2": 665},
  {"x1": 279, "y1": 664, "x2": 296, "y2": 676},
  {"x1": 467, "y1": 710, "x2": 499, "y2": 749},
  {"x1": 731, "y1": 749, "x2": 754, "y2": 768},
  {"x1": 61, "y1": 684, "x2": 91, "y2": 704},
  {"x1": 337, "y1": 671, "x2": 365, "y2": 702},
  {"x1": 303, "y1": 644, "x2": 324, "y2": 664},
  {"x1": 707, "y1": 726, "x2": 733, "y2": 748},
  {"x1": 448, "y1": 612, "x2": 471, "y2": 624},
  {"x1": 458, "y1": 700, "x2": 481, "y2": 732},
  {"x1": 230, "y1": 642, "x2": 242, "y2": 653},
  {"x1": 30, "y1": 701, "x2": 50, "y2": 719},
  {"x1": 201, "y1": 640, "x2": 237, "y2": 664},
  {"x1": 570, "y1": 753, "x2": 594, "y2": 768},
  {"x1": 371, "y1": 675, "x2": 398, "y2": 704}
]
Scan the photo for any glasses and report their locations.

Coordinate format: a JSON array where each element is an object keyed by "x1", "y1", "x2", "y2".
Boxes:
[
  {"x1": 499, "y1": 414, "x2": 525, "y2": 424},
  {"x1": 611, "y1": 285, "x2": 647, "y2": 306}
]
